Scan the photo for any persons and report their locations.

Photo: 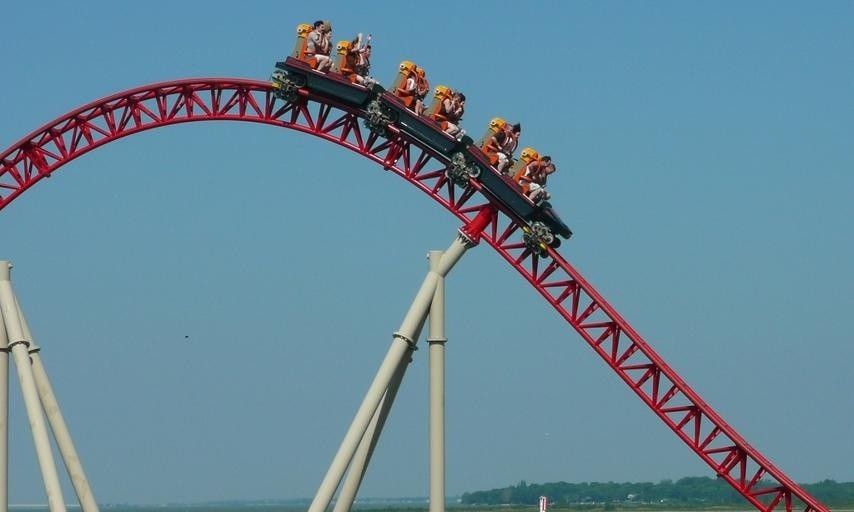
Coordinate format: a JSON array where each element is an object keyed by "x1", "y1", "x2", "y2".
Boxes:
[
  {"x1": 406, "y1": 69, "x2": 429, "y2": 116},
  {"x1": 491, "y1": 122, "x2": 521, "y2": 176},
  {"x1": 436, "y1": 91, "x2": 465, "y2": 139},
  {"x1": 519, "y1": 155, "x2": 556, "y2": 204},
  {"x1": 306, "y1": 20, "x2": 333, "y2": 75},
  {"x1": 345, "y1": 32, "x2": 380, "y2": 87}
]
